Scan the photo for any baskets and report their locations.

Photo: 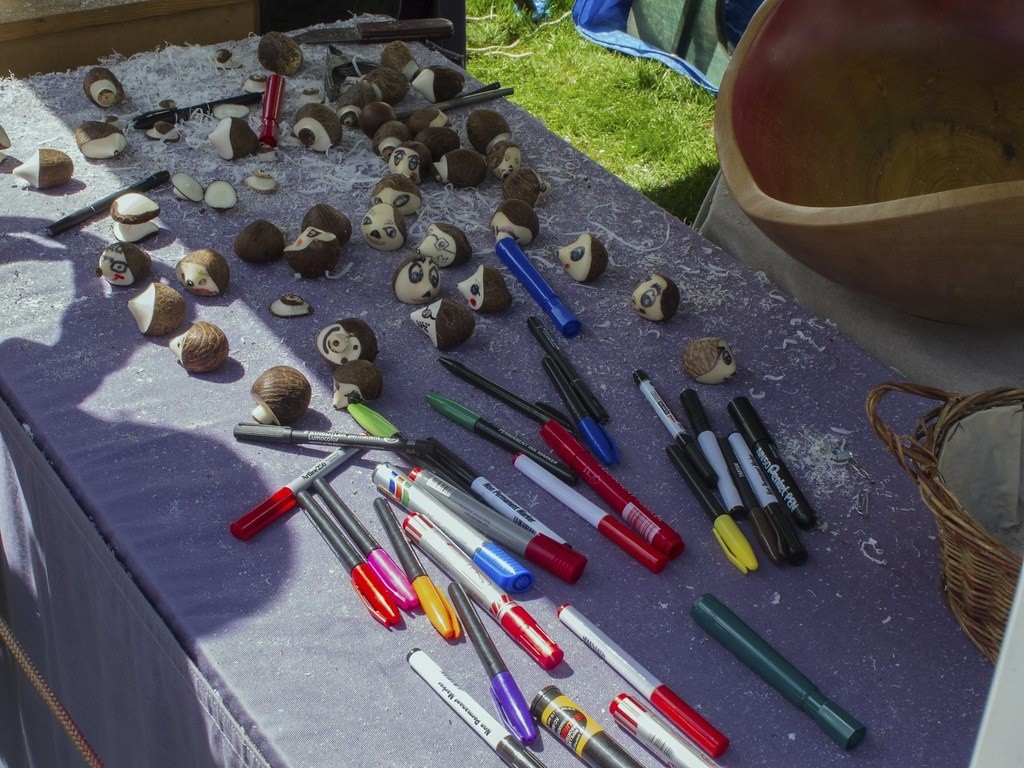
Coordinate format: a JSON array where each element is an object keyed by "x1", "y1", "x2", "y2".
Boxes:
[{"x1": 866, "y1": 380, "x2": 1024, "y2": 664}]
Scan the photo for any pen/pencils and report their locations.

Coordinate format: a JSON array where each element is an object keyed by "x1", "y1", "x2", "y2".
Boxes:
[
  {"x1": 224, "y1": 310, "x2": 874, "y2": 766},
  {"x1": 395, "y1": 82, "x2": 515, "y2": 124},
  {"x1": 44, "y1": 167, "x2": 171, "y2": 238},
  {"x1": 131, "y1": 90, "x2": 263, "y2": 131}
]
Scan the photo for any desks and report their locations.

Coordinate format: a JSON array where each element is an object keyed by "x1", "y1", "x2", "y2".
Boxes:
[{"x1": 0, "y1": 10, "x2": 1011, "y2": 768}]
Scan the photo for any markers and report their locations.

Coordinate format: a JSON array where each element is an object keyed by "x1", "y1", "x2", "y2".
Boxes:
[
  {"x1": 536, "y1": 418, "x2": 687, "y2": 560},
  {"x1": 401, "y1": 511, "x2": 565, "y2": 672},
  {"x1": 686, "y1": 591, "x2": 868, "y2": 752},
  {"x1": 493, "y1": 236, "x2": 584, "y2": 339},
  {"x1": 406, "y1": 465, "x2": 588, "y2": 587},
  {"x1": 369, "y1": 458, "x2": 537, "y2": 595},
  {"x1": 259, "y1": 73, "x2": 286, "y2": 148}
]
